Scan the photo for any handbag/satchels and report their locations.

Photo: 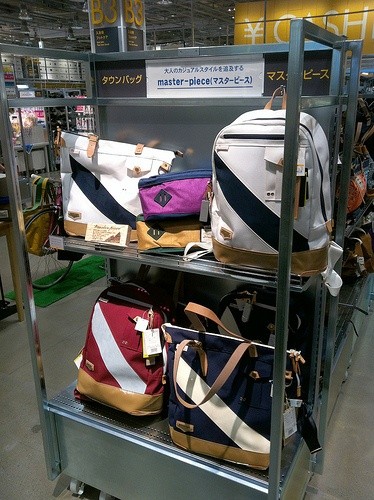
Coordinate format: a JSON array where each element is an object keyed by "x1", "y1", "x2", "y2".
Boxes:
[
  {"x1": 212, "y1": 282, "x2": 307, "y2": 391},
  {"x1": 137, "y1": 166, "x2": 218, "y2": 219},
  {"x1": 158, "y1": 301, "x2": 305, "y2": 472},
  {"x1": 132, "y1": 213, "x2": 213, "y2": 256},
  {"x1": 337, "y1": 117, "x2": 374, "y2": 280},
  {"x1": 58, "y1": 128, "x2": 181, "y2": 243},
  {"x1": 21, "y1": 176, "x2": 60, "y2": 255}
]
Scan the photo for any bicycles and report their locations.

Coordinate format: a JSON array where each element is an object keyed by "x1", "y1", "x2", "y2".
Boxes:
[{"x1": 22, "y1": 171, "x2": 84, "y2": 288}]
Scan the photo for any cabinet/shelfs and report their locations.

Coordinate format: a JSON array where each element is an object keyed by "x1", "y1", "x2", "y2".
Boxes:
[
  {"x1": 0, "y1": 17, "x2": 374, "y2": 500},
  {"x1": 46, "y1": 90, "x2": 94, "y2": 134}
]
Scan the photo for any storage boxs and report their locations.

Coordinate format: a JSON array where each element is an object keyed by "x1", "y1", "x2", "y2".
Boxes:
[{"x1": 1, "y1": 53, "x2": 85, "y2": 98}]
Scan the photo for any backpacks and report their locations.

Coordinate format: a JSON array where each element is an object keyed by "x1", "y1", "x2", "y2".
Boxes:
[
  {"x1": 76, "y1": 263, "x2": 178, "y2": 420},
  {"x1": 214, "y1": 81, "x2": 342, "y2": 297}
]
